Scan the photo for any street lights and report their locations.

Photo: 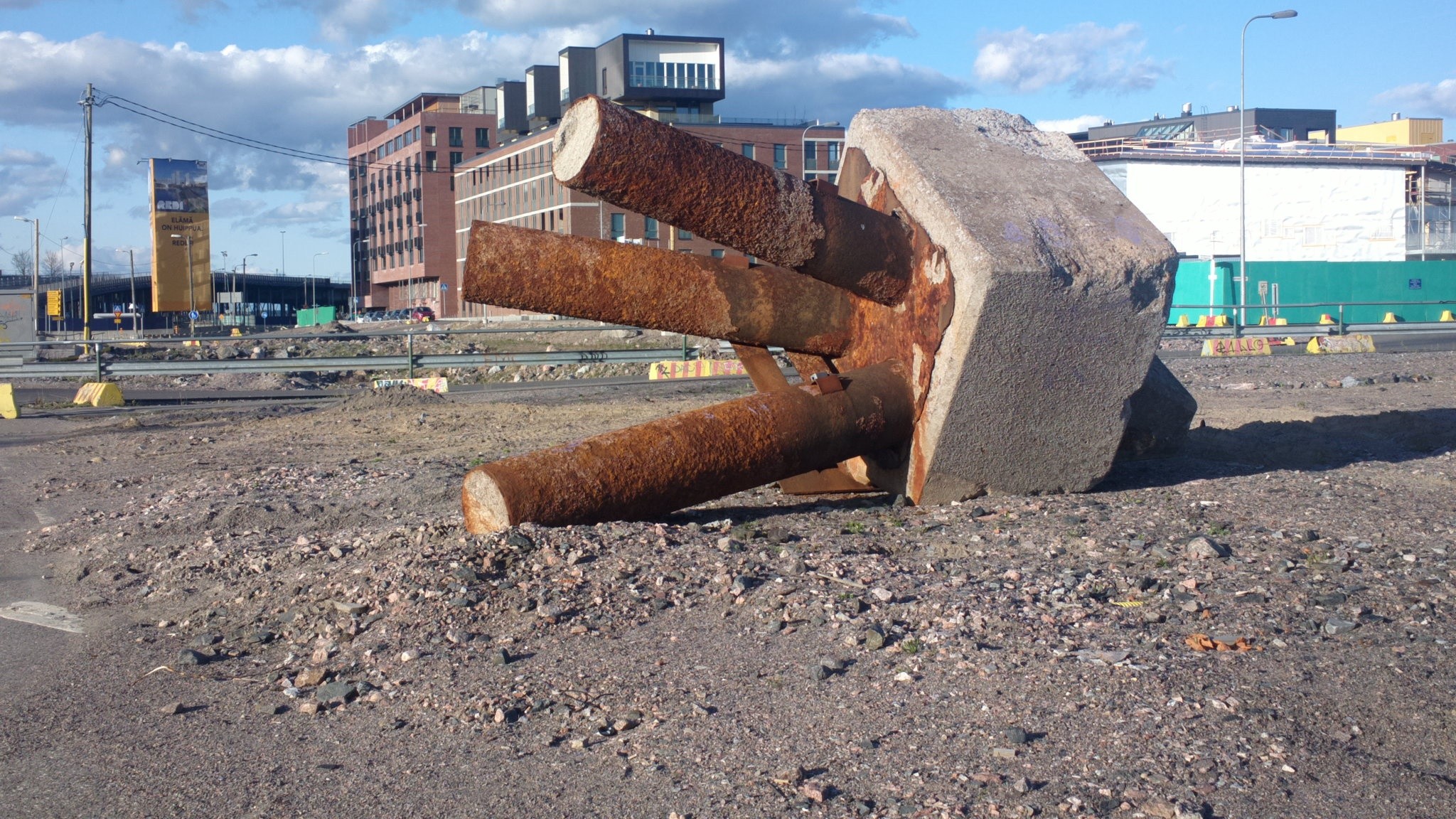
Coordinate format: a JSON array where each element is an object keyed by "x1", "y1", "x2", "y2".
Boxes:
[
  {"x1": 115, "y1": 248, "x2": 138, "y2": 337},
  {"x1": 243, "y1": 254, "x2": 258, "y2": 332},
  {"x1": 353, "y1": 239, "x2": 371, "y2": 322},
  {"x1": 171, "y1": 234, "x2": 195, "y2": 338},
  {"x1": 280, "y1": 230, "x2": 285, "y2": 275},
  {"x1": 13, "y1": 215, "x2": 39, "y2": 290},
  {"x1": 69, "y1": 262, "x2": 75, "y2": 320},
  {"x1": 481, "y1": 202, "x2": 507, "y2": 323},
  {"x1": 60, "y1": 236, "x2": 69, "y2": 340},
  {"x1": 79, "y1": 259, "x2": 86, "y2": 320},
  {"x1": 1242, "y1": 10, "x2": 1299, "y2": 322},
  {"x1": 210, "y1": 249, "x2": 247, "y2": 332},
  {"x1": 304, "y1": 274, "x2": 312, "y2": 307},
  {"x1": 313, "y1": 252, "x2": 330, "y2": 327},
  {"x1": 408, "y1": 223, "x2": 427, "y2": 319}
]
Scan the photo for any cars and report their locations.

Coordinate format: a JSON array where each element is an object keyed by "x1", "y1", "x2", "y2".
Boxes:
[{"x1": 337, "y1": 306, "x2": 437, "y2": 322}]
